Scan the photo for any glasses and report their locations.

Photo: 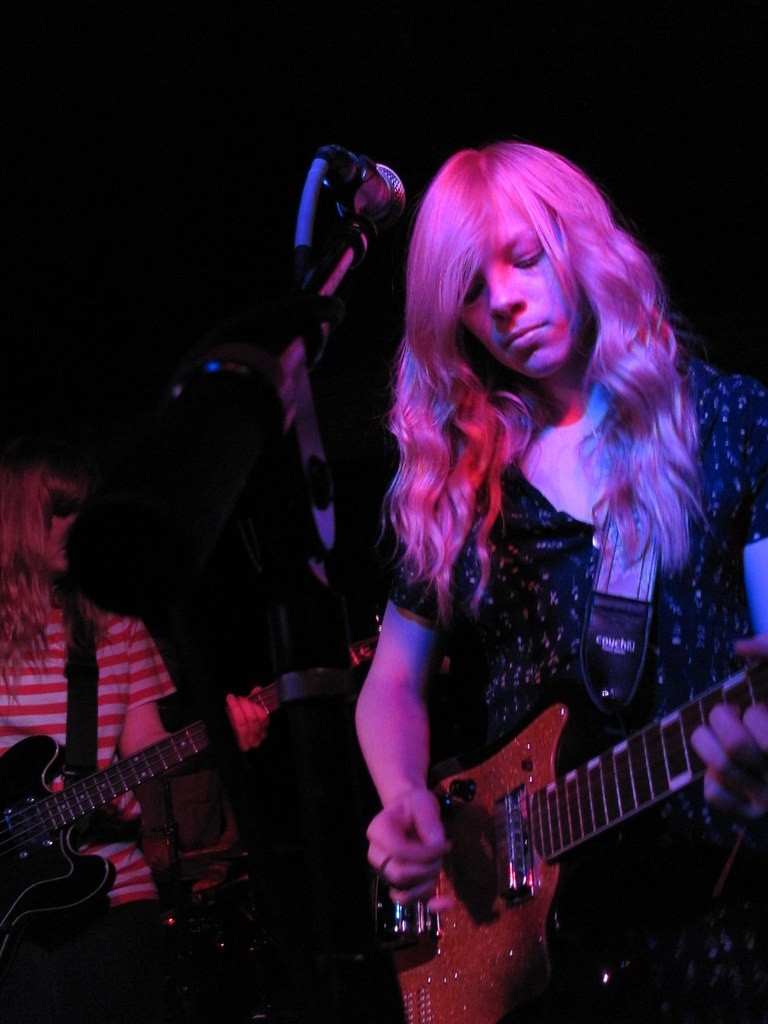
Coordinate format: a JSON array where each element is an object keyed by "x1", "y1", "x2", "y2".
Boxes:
[{"x1": 52, "y1": 494, "x2": 83, "y2": 517}]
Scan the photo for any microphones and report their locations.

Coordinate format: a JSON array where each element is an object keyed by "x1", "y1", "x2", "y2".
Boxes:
[{"x1": 317, "y1": 144, "x2": 406, "y2": 229}]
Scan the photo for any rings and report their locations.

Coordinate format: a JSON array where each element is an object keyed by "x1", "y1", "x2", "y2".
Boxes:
[{"x1": 379, "y1": 856, "x2": 391, "y2": 872}]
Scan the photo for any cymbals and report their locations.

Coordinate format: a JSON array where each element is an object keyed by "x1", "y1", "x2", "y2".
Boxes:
[{"x1": 177, "y1": 841, "x2": 263, "y2": 861}]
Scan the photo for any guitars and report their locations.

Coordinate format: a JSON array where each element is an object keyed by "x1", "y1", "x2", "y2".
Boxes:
[
  {"x1": 394, "y1": 654, "x2": 767, "y2": 1023},
  {"x1": 0, "y1": 678, "x2": 285, "y2": 974}
]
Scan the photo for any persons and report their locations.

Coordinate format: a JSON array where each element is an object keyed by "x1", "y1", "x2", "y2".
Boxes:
[
  {"x1": 352, "y1": 142, "x2": 768, "y2": 1024},
  {"x1": 0, "y1": 458, "x2": 271, "y2": 1024},
  {"x1": 136, "y1": 747, "x2": 240, "y2": 899}
]
